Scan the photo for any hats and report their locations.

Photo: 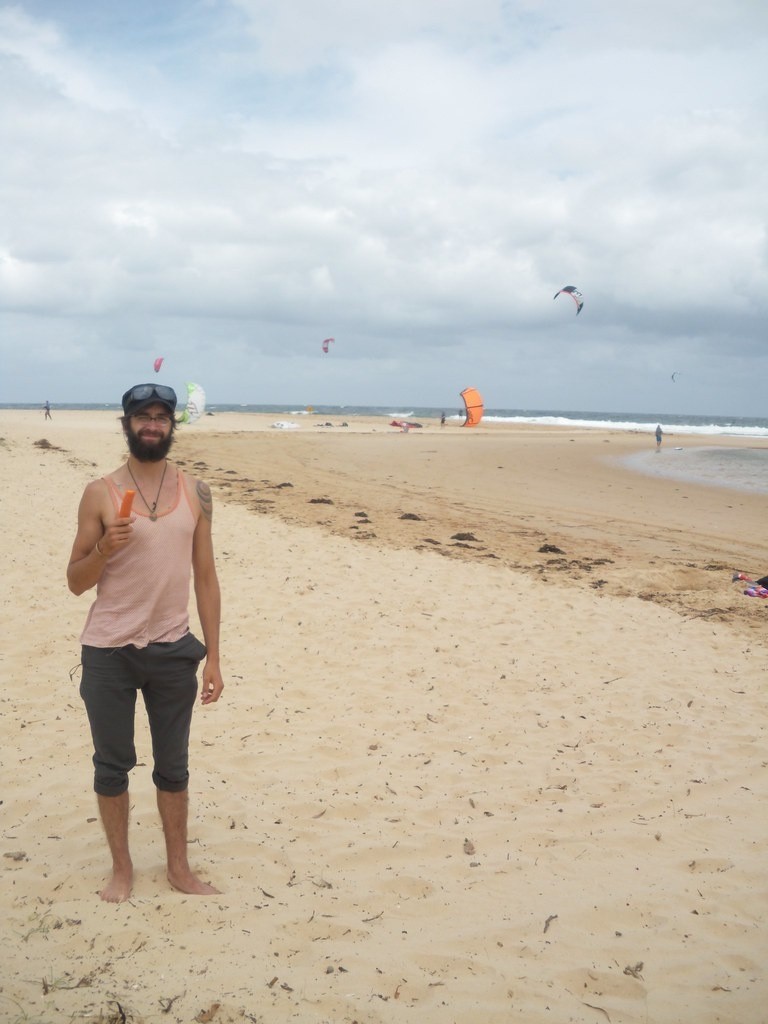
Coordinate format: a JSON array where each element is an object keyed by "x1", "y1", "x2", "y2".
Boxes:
[{"x1": 122, "y1": 382, "x2": 177, "y2": 417}]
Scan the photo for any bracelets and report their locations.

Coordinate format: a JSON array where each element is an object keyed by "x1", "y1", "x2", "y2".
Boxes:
[{"x1": 96, "y1": 544, "x2": 109, "y2": 558}]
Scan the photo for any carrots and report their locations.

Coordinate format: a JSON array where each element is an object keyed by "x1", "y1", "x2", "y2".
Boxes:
[{"x1": 119, "y1": 490, "x2": 136, "y2": 518}]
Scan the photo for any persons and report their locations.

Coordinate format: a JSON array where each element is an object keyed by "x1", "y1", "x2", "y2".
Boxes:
[
  {"x1": 655, "y1": 425, "x2": 663, "y2": 446},
  {"x1": 69, "y1": 384, "x2": 224, "y2": 904},
  {"x1": 45, "y1": 400, "x2": 51, "y2": 420},
  {"x1": 441, "y1": 409, "x2": 464, "y2": 426}
]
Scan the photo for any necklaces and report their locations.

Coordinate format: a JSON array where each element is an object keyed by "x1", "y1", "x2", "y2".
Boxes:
[{"x1": 128, "y1": 460, "x2": 168, "y2": 522}]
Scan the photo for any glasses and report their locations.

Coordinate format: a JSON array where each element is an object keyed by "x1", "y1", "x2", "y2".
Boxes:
[{"x1": 125, "y1": 385, "x2": 177, "y2": 412}]
